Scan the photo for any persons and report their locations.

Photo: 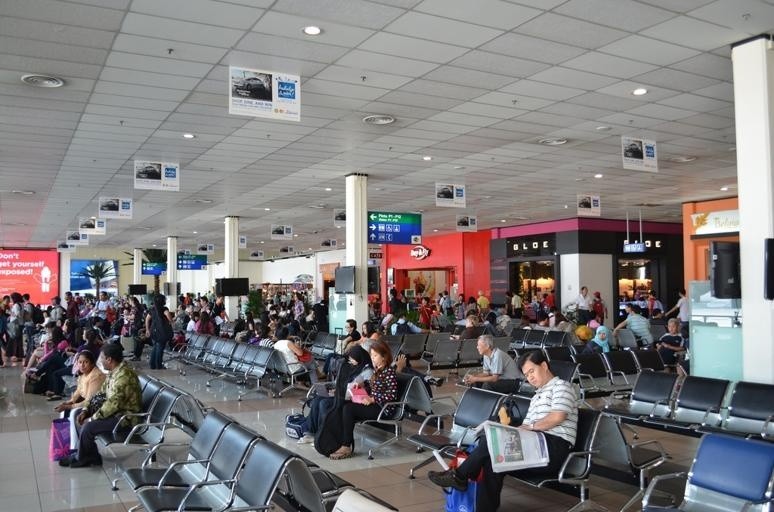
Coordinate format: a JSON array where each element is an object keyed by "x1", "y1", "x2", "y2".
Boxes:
[
  {"x1": 53, "y1": 350, "x2": 104, "y2": 456},
  {"x1": 58, "y1": 340, "x2": 145, "y2": 470},
  {"x1": 570, "y1": 286, "x2": 592, "y2": 323},
  {"x1": 591, "y1": 291, "x2": 609, "y2": 325},
  {"x1": 664, "y1": 288, "x2": 690, "y2": 338},
  {"x1": 652, "y1": 309, "x2": 663, "y2": 320},
  {"x1": 624, "y1": 303, "x2": 632, "y2": 318},
  {"x1": 654, "y1": 320, "x2": 684, "y2": 367},
  {"x1": 623, "y1": 291, "x2": 630, "y2": 302},
  {"x1": 580, "y1": 325, "x2": 610, "y2": 353},
  {"x1": 295, "y1": 344, "x2": 375, "y2": 447},
  {"x1": 328, "y1": 342, "x2": 399, "y2": 460},
  {"x1": 612, "y1": 305, "x2": 654, "y2": 349},
  {"x1": 426, "y1": 350, "x2": 579, "y2": 511},
  {"x1": 646, "y1": 290, "x2": 656, "y2": 315}
]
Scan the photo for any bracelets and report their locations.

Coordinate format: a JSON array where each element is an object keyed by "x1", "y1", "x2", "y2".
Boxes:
[{"x1": 528, "y1": 419, "x2": 537, "y2": 431}]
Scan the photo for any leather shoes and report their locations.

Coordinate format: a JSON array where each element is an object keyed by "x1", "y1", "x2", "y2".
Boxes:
[
  {"x1": 58, "y1": 454, "x2": 77, "y2": 467},
  {"x1": 69, "y1": 453, "x2": 101, "y2": 468}
]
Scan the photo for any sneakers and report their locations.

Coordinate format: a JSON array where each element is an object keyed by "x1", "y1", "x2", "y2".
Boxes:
[
  {"x1": 296, "y1": 435, "x2": 315, "y2": 443},
  {"x1": 424, "y1": 375, "x2": 443, "y2": 386},
  {"x1": 427, "y1": 466, "x2": 467, "y2": 492}
]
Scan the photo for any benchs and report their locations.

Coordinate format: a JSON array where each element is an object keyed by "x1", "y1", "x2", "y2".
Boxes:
[
  {"x1": 641, "y1": 431, "x2": 774, "y2": 512},
  {"x1": 112, "y1": 410, "x2": 401, "y2": 512},
  {"x1": 455, "y1": 328, "x2": 690, "y2": 411},
  {"x1": 62, "y1": 374, "x2": 80, "y2": 397},
  {"x1": 162, "y1": 328, "x2": 311, "y2": 401},
  {"x1": 307, "y1": 331, "x2": 348, "y2": 374},
  {"x1": 600, "y1": 370, "x2": 774, "y2": 443},
  {"x1": 360, "y1": 332, "x2": 462, "y2": 460},
  {"x1": 94, "y1": 371, "x2": 218, "y2": 477},
  {"x1": 406, "y1": 387, "x2": 673, "y2": 512},
  {"x1": 145, "y1": 344, "x2": 154, "y2": 359}
]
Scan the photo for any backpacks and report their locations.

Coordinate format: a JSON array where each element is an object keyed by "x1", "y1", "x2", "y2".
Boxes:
[
  {"x1": 496, "y1": 392, "x2": 521, "y2": 426},
  {"x1": 394, "y1": 320, "x2": 411, "y2": 335},
  {"x1": 26, "y1": 301, "x2": 43, "y2": 324}
]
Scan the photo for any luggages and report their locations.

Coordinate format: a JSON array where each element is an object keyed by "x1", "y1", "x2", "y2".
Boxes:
[
  {"x1": 327, "y1": 327, "x2": 346, "y2": 380},
  {"x1": 119, "y1": 326, "x2": 134, "y2": 358}
]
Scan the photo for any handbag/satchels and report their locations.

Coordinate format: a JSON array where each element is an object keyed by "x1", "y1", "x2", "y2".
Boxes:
[
  {"x1": 444, "y1": 449, "x2": 485, "y2": 511},
  {"x1": 284, "y1": 413, "x2": 309, "y2": 439},
  {"x1": 89, "y1": 393, "x2": 107, "y2": 416},
  {"x1": 296, "y1": 350, "x2": 312, "y2": 363},
  {"x1": 48, "y1": 417, "x2": 72, "y2": 461}
]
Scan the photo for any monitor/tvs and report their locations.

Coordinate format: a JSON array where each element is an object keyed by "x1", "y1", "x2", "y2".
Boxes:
[
  {"x1": 216, "y1": 278, "x2": 249, "y2": 296},
  {"x1": 334, "y1": 267, "x2": 356, "y2": 294},
  {"x1": 368, "y1": 266, "x2": 380, "y2": 294},
  {"x1": 710, "y1": 241, "x2": 741, "y2": 299},
  {"x1": 764, "y1": 239, "x2": 773, "y2": 300},
  {"x1": 164, "y1": 283, "x2": 181, "y2": 296},
  {"x1": 128, "y1": 284, "x2": 146, "y2": 294}
]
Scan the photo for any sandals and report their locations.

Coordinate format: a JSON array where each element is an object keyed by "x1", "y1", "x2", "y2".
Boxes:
[{"x1": 328, "y1": 447, "x2": 352, "y2": 460}]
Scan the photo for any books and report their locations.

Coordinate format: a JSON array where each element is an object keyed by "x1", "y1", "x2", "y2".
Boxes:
[{"x1": 472, "y1": 415, "x2": 550, "y2": 476}]
